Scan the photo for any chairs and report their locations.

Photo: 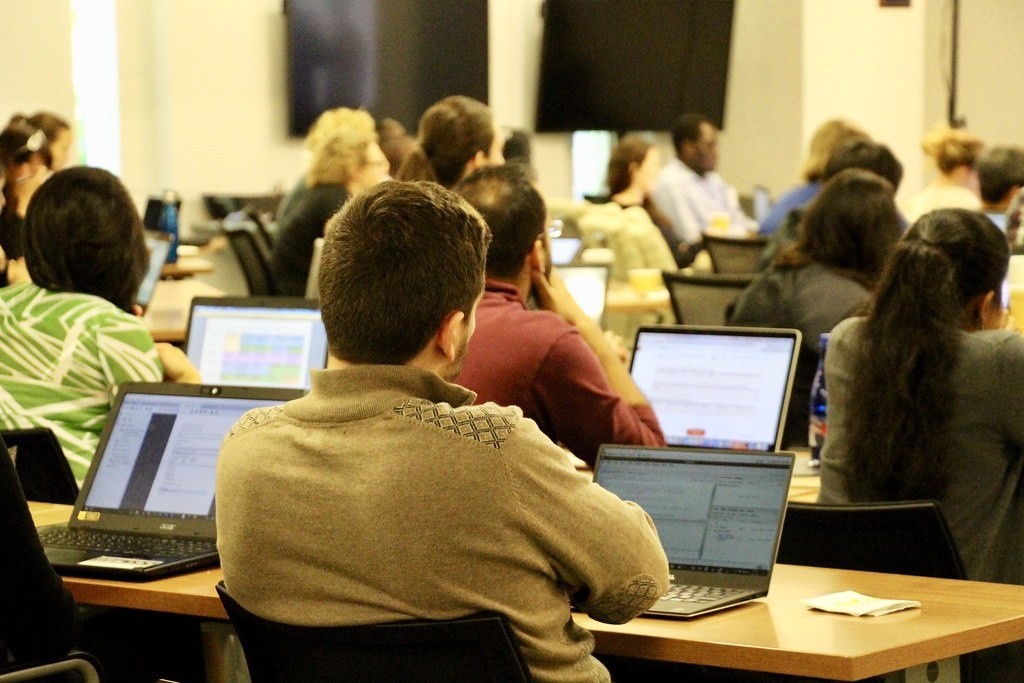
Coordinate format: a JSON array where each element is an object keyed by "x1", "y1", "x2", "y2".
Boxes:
[
  {"x1": 701, "y1": 233, "x2": 788, "y2": 277},
  {"x1": 774, "y1": 502, "x2": 966, "y2": 578},
  {"x1": 205, "y1": 196, "x2": 289, "y2": 300},
  {"x1": 0, "y1": 648, "x2": 105, "y2": 683},
  {"x1": 215, "y1": 580, "x2": 533, "y2": 683},
  {"x1": 0, "y1": 426, "x2": 80, "y2": 506},
  {"x1": 660, "y1": 269, "x2": 762, "y2": 329}
]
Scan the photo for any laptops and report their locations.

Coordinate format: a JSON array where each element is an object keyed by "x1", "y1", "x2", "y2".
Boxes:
[
  {"x1": 589, "y1": 443, "x2": 796, "y2": 618},
  {"x1": 34, "y1": 225, "x2": 332, "y2": 582},
  {"x1": 548, "y1": 239, "x2": 611, "y2": 333},
  {"x1": 628, "y1": 324, "x2": 803, "y2": 453}
]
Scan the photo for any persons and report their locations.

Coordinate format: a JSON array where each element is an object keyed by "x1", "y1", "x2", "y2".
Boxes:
[
  {"x1": 0, "y1": 112, "x2": 71, "y2": 285},
  {"x1": 759, "y1": 121, "x2": 875, "y2": 234},
  {"x1": 760, "y1": 136, "x2": 903, "y2": 283},
  {"x1": 0, "y1": 165, "x2": 203, "y2": 489},
  {"x1": 214, "y1": 181, "x2": 670, "y2": 683},
  {"x1": 396, "y1": 95, "x2": 505, "y2": 190},
  {"x1": 585, "y1": 135, "x2": 714, "y2": 274},
  {"x1": 909, "y1": 129, "x2": 985, "y2": 223},
  {"x1": 820, "y1": 213, "x2": 1024, "y2": 683},
  {"x1": 377, "y1": 119, "x2": 417, "y2": 178},
  {"x1": 501, "y1": 129, "x2": 531, "y2": 172},
  {"x1": 454, "y1": 165, "x2": 665, "y2": 470},
  {"x1": 651, "y1": 113, "x2": 758, "y2": 244},
  {"x1": 727, "y1": 170, "x2": 905, "y2": 448},
  {"x1": 268, "y1": 106, "x2": 390, "y2": 298},
  {"x1": 979, "y1": 145, "x2": 1024, "y2": 234}
]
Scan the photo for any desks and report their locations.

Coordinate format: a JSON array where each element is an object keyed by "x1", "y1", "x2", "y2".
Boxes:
[{"x1": 30, "y1": 500, "x2": 1024, "y2": 683}]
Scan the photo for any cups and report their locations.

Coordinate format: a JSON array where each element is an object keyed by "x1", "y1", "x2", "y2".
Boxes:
[
  {"x1": 625, "y1": 268, "x2": 661, "y2": 297},
  {"x1": 711, "y1": 212, "x2": 732, "y2": 235},
  {"x1": 1009, "y1": 283, "x2": 1023, "y2": 332}
]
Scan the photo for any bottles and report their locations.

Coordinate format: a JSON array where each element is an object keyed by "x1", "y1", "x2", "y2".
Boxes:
[
  {"x1": 157, "y1": 188, "x2": 177, "y2": 262},
  {"x1": 809, "y1": 332, "x2": 831, "y2": 468}
]
